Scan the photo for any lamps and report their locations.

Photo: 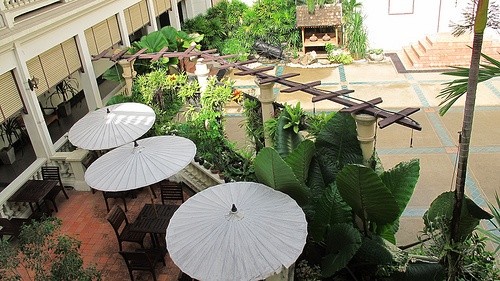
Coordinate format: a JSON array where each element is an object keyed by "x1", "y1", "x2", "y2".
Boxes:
[{"x1": 28, "y1": 75, "x2": 39, "y2": 91}]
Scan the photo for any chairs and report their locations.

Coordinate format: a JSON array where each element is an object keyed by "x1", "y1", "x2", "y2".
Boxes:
[
  {"x1": 39, "y1": 102, "x2": 60, "y2": 127},
  {"x1": 40, "y1": 166, "x2": 69, "y2": 212},
  {"x1": 106, "y1": 203, "x2": 155, "y2": 251},
  {"x1": 82, "y1": 150, "x2": 99, "y2": 194},
  {"x1": 0, "y1": 218, "x2": 30, "y2": 242},
  {"x1": 119, "y1": 249, "x2": 166, "y2": 281},
  {"x1": 161, "y1": 181, "x2": 184, "y2": 205},
  {"x1": 102, "y1": 190, "x2": 134, "y2": 213}
]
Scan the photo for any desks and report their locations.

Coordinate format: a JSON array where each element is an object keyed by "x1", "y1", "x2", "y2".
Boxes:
[
  {"x1": 130, "y1": 203, "x2": 180, "y2": 247},
  {"x1": 7, "y1": 180, "x2": 57, "y2": 222}
]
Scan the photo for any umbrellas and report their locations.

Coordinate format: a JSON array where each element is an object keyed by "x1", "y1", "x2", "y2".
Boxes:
[
  {"x1": 165, "y1": 181, "x2": 308, "y2": 281},
  {"x1": 67, "y1": 102, "x2": 156, "y2": 150},
  {"x1": 84, "y1": 136, "x2": 197, "y2": 217}
]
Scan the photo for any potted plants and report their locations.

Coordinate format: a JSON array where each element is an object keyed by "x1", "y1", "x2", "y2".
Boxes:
[
  {"x1": 177, "y1": 105, "x2": 243, "y2": 183},
  {"x1": 47, "y1": 77, "x2": 79, "y2": 119},
  {"x1": 344, "y1": 12, "x2": 370, "y2": 60},
  {"x1": 0, "y1": 117, "x2": 22, "y2": 165},
  {"x1": 370, "y1": 49, "x2": 384, "y2": 60}
]
